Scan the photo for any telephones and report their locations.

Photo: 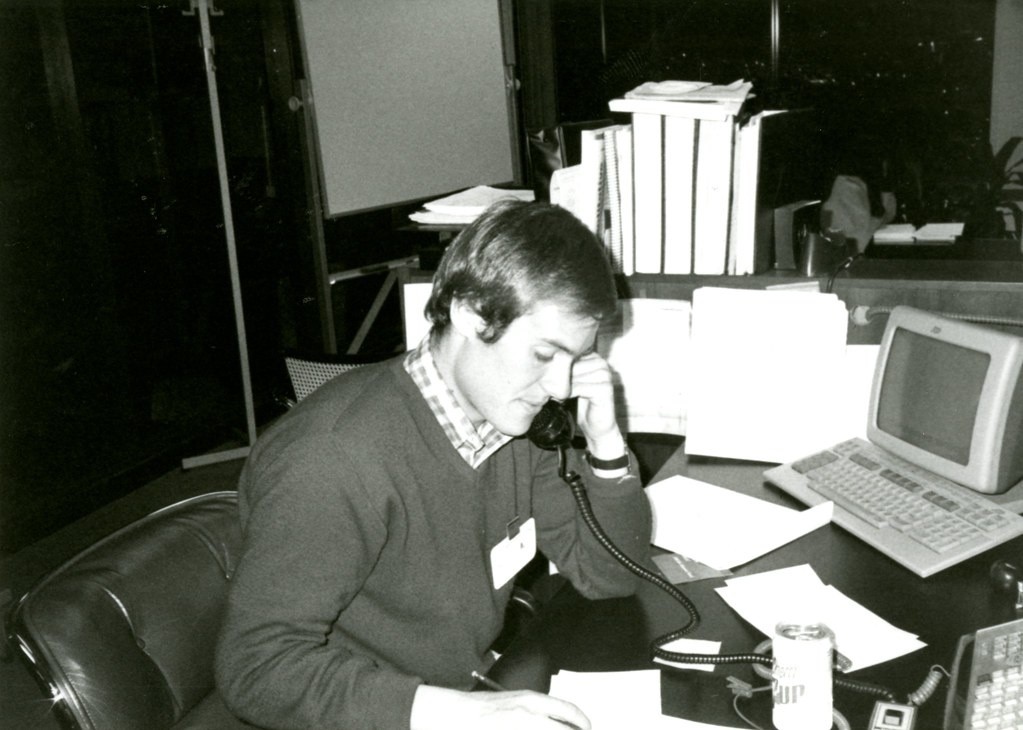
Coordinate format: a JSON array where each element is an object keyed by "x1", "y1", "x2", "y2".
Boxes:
[{"x1": 528, "y1": 399, "x2": 578, "y2": 453}]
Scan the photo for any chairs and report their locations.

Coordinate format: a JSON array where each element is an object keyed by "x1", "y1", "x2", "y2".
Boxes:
[{"x1": 20, "y1": 492, "x2": 266, "y2": 730}]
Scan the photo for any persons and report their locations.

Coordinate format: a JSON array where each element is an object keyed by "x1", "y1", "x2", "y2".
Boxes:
[{"x1": 213, "y1": 198, "x2": 653, "y2": 730}]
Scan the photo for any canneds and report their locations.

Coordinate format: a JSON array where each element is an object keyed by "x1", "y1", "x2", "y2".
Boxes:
[{"x1": 771, "y1": 619, "x2": 836, "y2": 729}]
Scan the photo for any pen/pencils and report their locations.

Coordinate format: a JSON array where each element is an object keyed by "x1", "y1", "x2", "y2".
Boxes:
[{"x1": 468, "y1": 669, "x2": 584, "y2": 730}]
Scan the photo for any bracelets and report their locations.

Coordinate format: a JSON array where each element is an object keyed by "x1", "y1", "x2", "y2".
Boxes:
[{"x1": 584, "y1": 445, "x2": 630, "y2": 469}]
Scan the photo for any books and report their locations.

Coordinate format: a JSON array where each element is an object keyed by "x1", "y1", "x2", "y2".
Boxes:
[{"x1": 547, "y1": 78, "x2": 788, "y2": 278}]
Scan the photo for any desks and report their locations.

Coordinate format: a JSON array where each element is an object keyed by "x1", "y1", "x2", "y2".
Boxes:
[{"x1": 473, "y1": 438, "x2": 1023, "y2": 730}]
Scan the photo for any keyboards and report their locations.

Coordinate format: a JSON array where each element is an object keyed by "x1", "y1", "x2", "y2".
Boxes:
[{"x1": 761, "y1": 435, "x2": 1023, "y2": 579}]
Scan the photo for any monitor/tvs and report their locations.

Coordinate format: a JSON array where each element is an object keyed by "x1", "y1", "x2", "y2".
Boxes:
[{"x1": 867, "y1": 304, "x2": 1023, "y2": 499}]
